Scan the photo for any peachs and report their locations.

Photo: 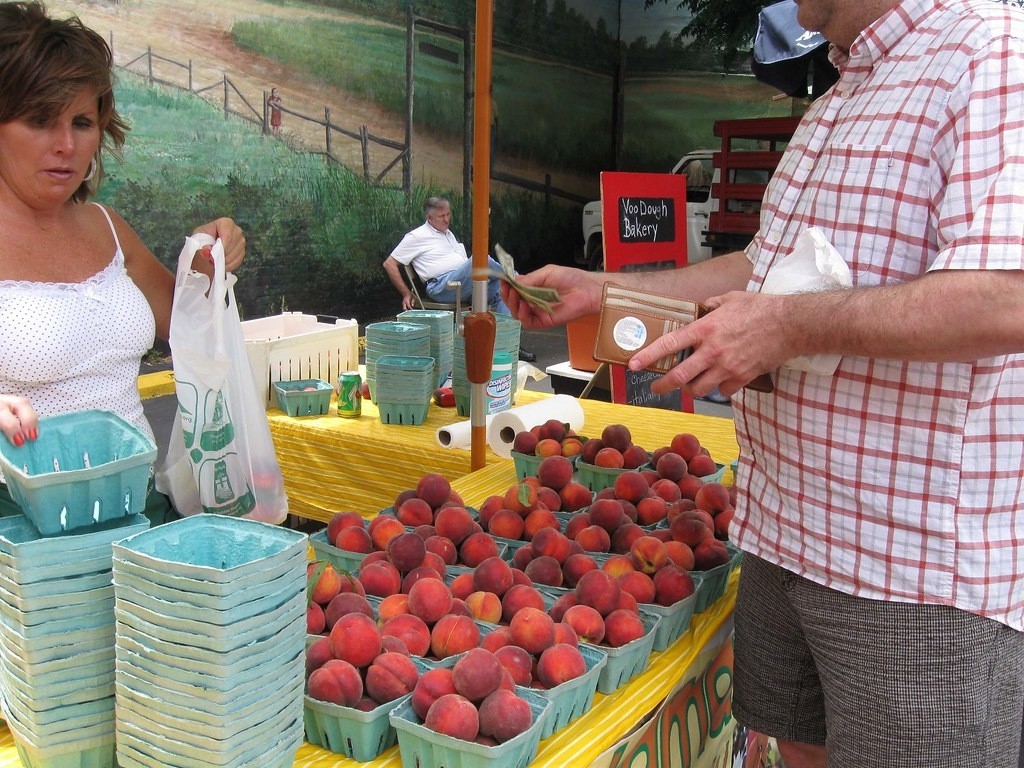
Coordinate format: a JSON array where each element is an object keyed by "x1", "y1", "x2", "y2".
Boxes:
[
  {"x1": 211, "y1": 460, "x2": 286, "y2": 525},
  {"x1": 299, "y1": 419, "x2": 736, "y2": 748},
  {"x1": 362, "y1": 382, "x2": 371, "y2": 400}
]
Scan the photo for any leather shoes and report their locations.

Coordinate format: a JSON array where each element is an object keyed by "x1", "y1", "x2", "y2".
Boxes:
[{"x1": 518, "y1": 347, "x2": 536, "y2": 361}]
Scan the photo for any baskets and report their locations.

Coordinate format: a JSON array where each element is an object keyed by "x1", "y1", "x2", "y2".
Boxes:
[
  {"x1": 366, "y1": 310, "x2": 521, "y2": 425},
  {"x1": 0, "y1": 512, "x2": 310, "y2": 768},
  {"x1": 273, "y1": 380, "x2": 333, "y2": 416},
  {"x1": 304, "y1": 436, "x2": 740, "y2": 768},
  {"x1": 0, "y1": 410, "x2": 159, "y2": 536}
]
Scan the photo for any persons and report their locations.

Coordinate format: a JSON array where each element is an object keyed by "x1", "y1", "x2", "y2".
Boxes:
[
  {"x1": 383, "y1": 195, "x2": 537, "y2": 363},
  {"x1": 0, "y1": 0, "x2": 247, "y2": 531},
  {"x1": 500, "y1": 0, "x2": 1024, "y2": 768}
]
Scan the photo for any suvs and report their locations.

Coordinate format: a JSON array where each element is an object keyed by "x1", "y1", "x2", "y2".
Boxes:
[{"x1": 580, "y1": 144, "x2": 779, "y2": 276}]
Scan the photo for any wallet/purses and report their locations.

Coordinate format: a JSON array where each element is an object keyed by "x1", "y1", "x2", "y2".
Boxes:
[{"x1": 592, "y1": 281, "x2": 774, "y2": 394}]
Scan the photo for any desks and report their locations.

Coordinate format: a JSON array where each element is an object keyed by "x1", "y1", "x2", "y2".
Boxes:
[
  {"x1": 0, "y1": 461, "x2": 788, "y2": 768},
  {"x1": 268, "y1": 365, "x2": 743, "y2": 531}
]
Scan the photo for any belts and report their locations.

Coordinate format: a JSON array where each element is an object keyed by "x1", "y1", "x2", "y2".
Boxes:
[{"x1": 423, "y1": 278, "x2": 434, "y2": 287}]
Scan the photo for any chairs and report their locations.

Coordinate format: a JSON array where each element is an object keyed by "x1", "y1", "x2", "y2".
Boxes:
[{"x1": 404, "y1": 264, "x2": 471, "y2": 314}]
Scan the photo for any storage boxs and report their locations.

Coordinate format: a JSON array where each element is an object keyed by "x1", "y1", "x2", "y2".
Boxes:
[
  {"x1": 1, "y1": 408, "x2": 745, "y2": 768},
  {"x1": 234, "y1": 311, "x2": 358, "y2": 416},
  {"x1": 365, "y1": 310, "x2": 521, "y2": 425}
]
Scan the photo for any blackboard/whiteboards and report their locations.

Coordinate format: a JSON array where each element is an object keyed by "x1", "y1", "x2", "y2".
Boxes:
[{"x1": 600, "y1": 171, "x2": 695, "y2": 413}]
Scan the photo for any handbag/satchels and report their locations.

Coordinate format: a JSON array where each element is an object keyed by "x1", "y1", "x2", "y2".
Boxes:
[{"x1": 154, "y1": 232, "x2": 289, "y2": 525}]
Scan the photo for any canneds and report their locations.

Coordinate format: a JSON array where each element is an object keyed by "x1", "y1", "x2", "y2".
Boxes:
[{"x1": 337, "y1": 371, "x2": 362, "y2": 418}]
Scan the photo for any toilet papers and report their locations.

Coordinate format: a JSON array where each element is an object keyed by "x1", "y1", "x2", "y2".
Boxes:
[
  {"x1": 488, "y1": 394, "x2": 585, "y2": 458},
  {"x1": 436, "y1": 413, "x2": 497, "y2": 450}
]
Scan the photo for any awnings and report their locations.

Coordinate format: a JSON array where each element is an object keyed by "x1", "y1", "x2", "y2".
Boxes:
[{"x1": 751, "y1": 0, "x2": 840, "y2": 101}]
